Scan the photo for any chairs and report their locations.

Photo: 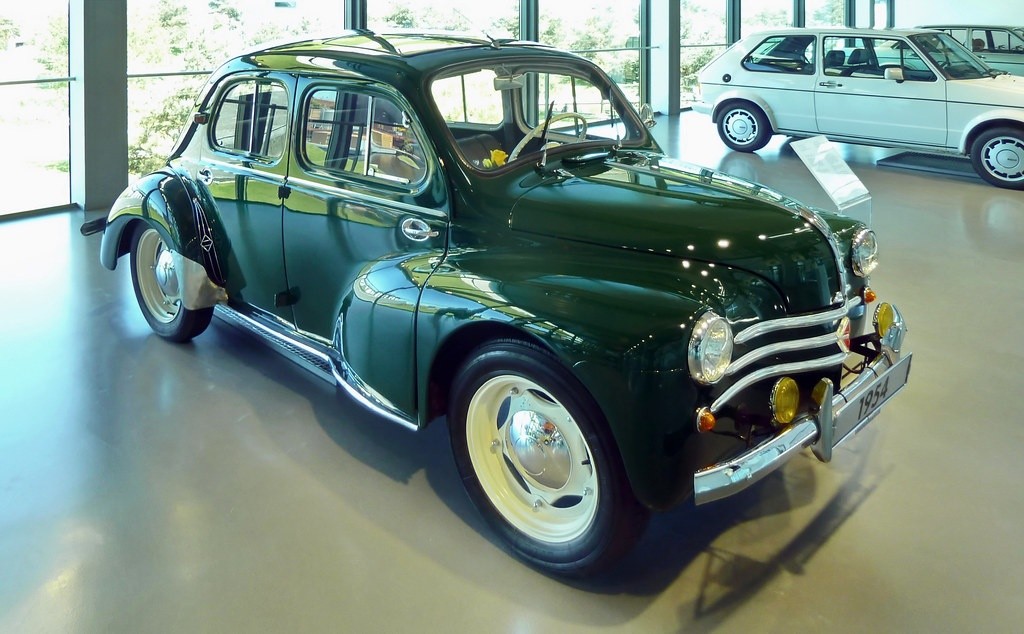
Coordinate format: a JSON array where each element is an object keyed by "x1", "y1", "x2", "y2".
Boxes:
[
  {"x1": 823, "y1": 48, "x2": 878, "y2": 76},
  {"x1": 962, "y1": 38, "x2": 986, "y2": 52},
  {"x1": 367, "y1": 133, "x2": 511, "y2": 183}
]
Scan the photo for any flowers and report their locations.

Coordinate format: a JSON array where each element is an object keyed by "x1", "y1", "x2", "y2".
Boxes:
[{"x1": 471, "y1": 149, "x2": 509, "y2": 170}]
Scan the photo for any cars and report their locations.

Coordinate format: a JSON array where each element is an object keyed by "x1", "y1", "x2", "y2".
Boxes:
[
  {"x1": 80, "y1": 27, "x2": 910, "y2": 584},
  {"x1": 683, "y1": 28, "x2": 1023, "y2": 190},
  {"x1": 833, "y1": 23, "x2": 1023, "y2": 78}
]
